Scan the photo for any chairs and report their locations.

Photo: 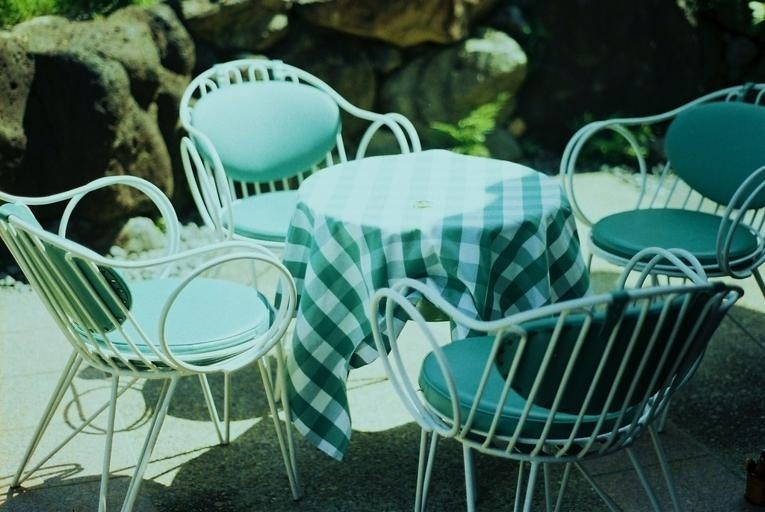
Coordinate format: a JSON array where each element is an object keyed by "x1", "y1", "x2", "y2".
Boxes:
[
  {"x1": 0, "y1": 176, "x2": 305, "y2": 510},
  {"x1": 175, "y1": 53, "x2": 421, "y2": 403},
  {"x1": 558, "y1": 78, "x2": 763, "y2": 433},
  {"x1": 364, "y1": 249, "x2": 744, "y2": 511}
]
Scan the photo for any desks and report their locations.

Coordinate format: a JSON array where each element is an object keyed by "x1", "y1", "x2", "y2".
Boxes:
[{"x1": 295, "y1": 153, "x2": 572, "y2": 510}]
